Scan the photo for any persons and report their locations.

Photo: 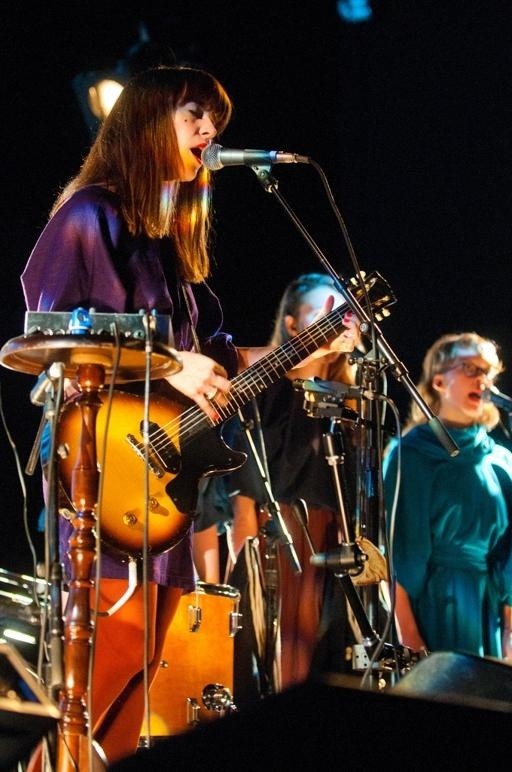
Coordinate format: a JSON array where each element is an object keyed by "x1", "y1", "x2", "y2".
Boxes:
[
  {"x1": 15, "y1": 57, "x2": 363, "y2": 772},
  {"x1": 30, "y1": 358, "x2": 231, "y2": 618},
  {"x1": 230, "y1": 260, "x2": 373, "y2": 704},
  {"x1": 358, "y1": 325, "x2": 511, "y2": 653}
]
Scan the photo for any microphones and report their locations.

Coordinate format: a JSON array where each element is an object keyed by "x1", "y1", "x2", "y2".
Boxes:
[
  {"x1": 200, "y1": 680, "x2": 240, "y2": 716},
  {"x1": 292, "y1": 376, "x2": 378, "y2": 404},
  {"x1": 201, "y1": 143, "x2": 311, "y2": 172},
  {"x1": 480, "y1": 386, "x2": 512, "y2": 414},
  {"x1": 29, "y1": 362, "x2": 69, "y2": 408}
]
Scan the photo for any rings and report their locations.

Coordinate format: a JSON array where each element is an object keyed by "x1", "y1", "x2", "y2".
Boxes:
[{"x1": 206, "y1": 388, "x2": 223, "y2": 401}]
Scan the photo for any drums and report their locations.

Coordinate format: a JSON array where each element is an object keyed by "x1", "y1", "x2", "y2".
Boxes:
[{"x1": 140, "y1": 580, "x2": 244, "y2": 741}]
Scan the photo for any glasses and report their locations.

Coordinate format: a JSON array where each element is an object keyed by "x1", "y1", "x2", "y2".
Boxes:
[{"x1": 440, "y1": 361, "x2": 487, "y2": 377}]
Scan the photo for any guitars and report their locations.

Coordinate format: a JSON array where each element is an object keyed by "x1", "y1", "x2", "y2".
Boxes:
[{"x1": 51, "y1": 270, "x2": 398, "y2": 561}]
